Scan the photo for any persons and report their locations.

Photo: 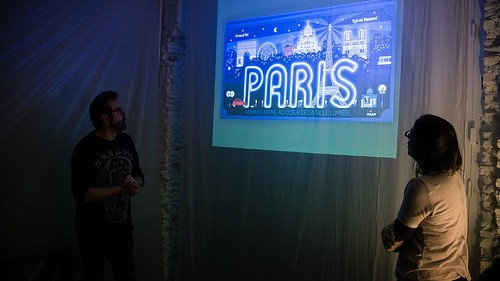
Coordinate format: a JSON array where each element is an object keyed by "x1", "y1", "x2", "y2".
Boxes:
[
  {"x1": 70, "y1": 90, "x2": 145, "y2": 281},
  {"x1": 380, "y1": 114, "x2": 472, "y2": 281}
]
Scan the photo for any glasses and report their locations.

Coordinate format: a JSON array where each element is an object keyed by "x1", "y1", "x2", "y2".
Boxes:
[
  {"x1": 403, "y1": 129, "x2": 412, "y2": 139},
  {"x1": 112, "y1": 107, "x2": 124, "y2": 113}
]
